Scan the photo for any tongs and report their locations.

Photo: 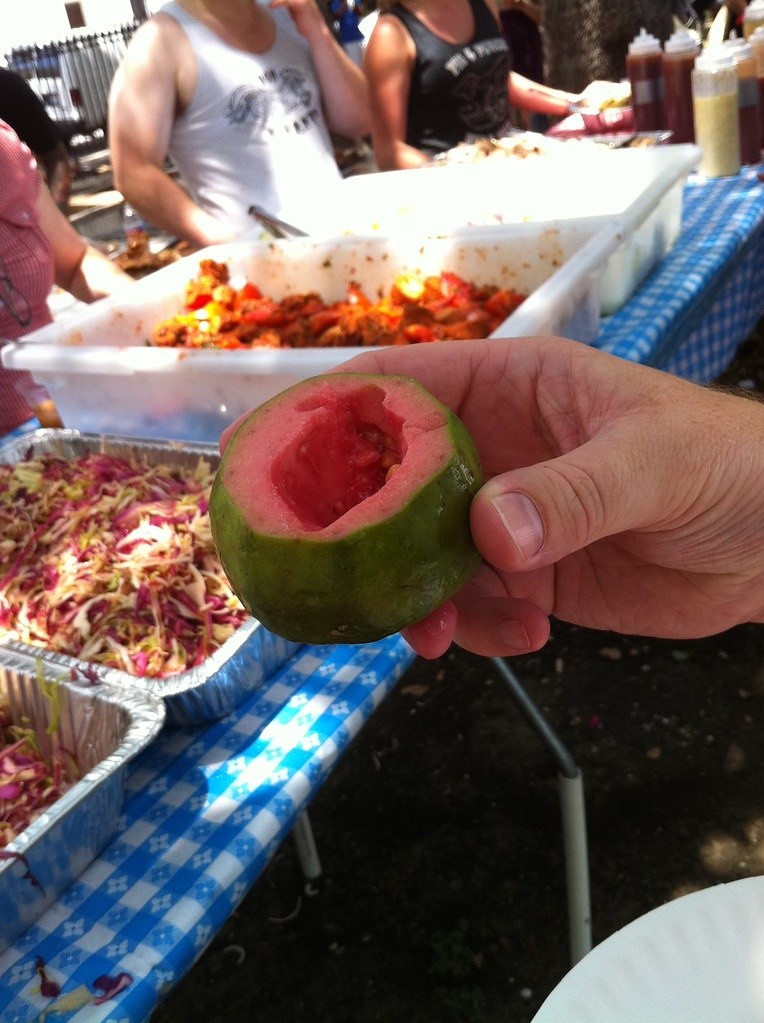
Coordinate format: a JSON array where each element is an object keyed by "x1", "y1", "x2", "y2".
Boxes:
[{"x1": 248, "y1": 206, "x2": 310, "y2": 239}]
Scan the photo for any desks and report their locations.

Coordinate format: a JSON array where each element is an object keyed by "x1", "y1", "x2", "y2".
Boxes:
[
  {"x1": 535, "y1": 873, "x2": 764, "y2": 1023},
  {"x1": 0, "y1": 166, "x2": 764, "y2": 1023}
]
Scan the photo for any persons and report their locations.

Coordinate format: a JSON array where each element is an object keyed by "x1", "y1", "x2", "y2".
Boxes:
[
  {"x1": 218, "y1": 334, "x2": 764, "y2": 662},
  {"x1": 0, "y1": 120, "x2": 142, "y2": 436},
  {"x1": 319, "y1": 0, "x2": 548, "y2": 133},
  {"x1": 0, "y1": 68, "x2": 60, "y2": 186},
  {"x1": 363, "y1": 0, "x2": 578, "y2": 170},
  {"x1": 108, "y1": 0, "x2": 372, "y2": 250}
]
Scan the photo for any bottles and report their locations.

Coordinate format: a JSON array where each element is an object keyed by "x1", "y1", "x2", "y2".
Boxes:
[
  {"x1": 123, "y1": 200, "x2": 146, "y2": 246},
  {"x1": 661, "y1": 28, "x2": 699, "y2": 143},
  {"x1": 691, "y1": 39, "x2": 739, "y2": 177},
  {"x1": 626, "y1": 27, "x2": 664, "y2": 132},
  {"x1": 724, "y1": 30, "x2": 761, "y2": 165},
  {"x1": 743, "y1": 0, "x2": 764, "y2": 44},
  {"x1": 747, "y1": 25, "x2": 764, "y2": 152}
]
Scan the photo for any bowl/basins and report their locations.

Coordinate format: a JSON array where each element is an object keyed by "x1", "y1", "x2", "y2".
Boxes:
[{"x1": 571, "y1": 101, "x2": 633, "y2": 133}]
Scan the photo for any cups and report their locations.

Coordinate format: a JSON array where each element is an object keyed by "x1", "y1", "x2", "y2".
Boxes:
[{"x1": 13, "y1": 376, "x2": 64, "y2": 429}]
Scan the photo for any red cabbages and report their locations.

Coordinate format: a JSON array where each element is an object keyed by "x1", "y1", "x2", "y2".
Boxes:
[{"x1": 0, "y1": 442, "x2": 246, "y2": 855}]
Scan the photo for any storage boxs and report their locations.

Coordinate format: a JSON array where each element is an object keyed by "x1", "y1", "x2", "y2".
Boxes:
[
  {"x1": 240, "y1": 143, "x2": 700, "y2": 314},
  {"x1": 0, "y1": 234, "x2": 620, "y2": 449},
  {"x1": 0, "y1": 650, "x2": 167, "y2": 954},
  {"x1": 0, "y1": 429, "x2": 302, "y2": 724}
]
